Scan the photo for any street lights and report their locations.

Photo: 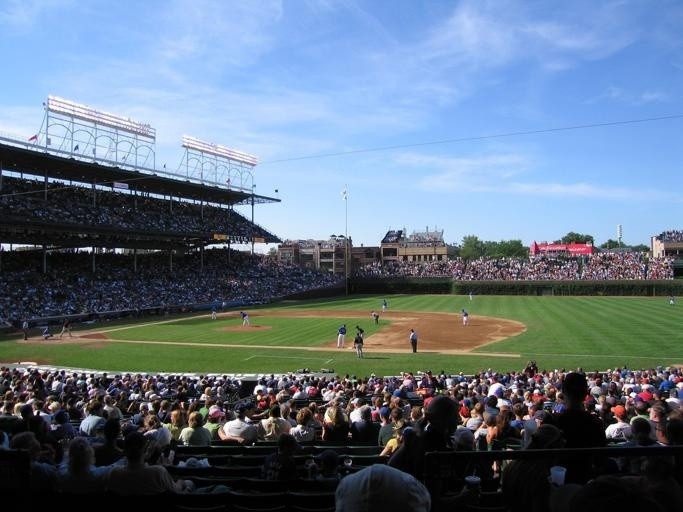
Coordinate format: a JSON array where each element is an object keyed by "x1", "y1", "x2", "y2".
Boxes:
[
  {"x1": 342, "y1": 182, "x2": 351, "y2": 294},
  {"x1": 617, "y1": 222, "x2": 624, "y2": 254}
]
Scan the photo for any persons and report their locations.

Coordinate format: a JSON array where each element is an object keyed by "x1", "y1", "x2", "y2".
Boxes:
[
  {"x1": 356, "y1": 326, "x2": 364, "y2": 339},
  {"x1": 353, "y1": 333, "x2": 363, "y2": 358},
  {"x1": 42, "y1": 327, "x2": 53, "y2": 339},
  {"x1": 60, "y1": 319, "x2": 73, "y2": 337},
  {"x1": 410, "y1": 329, "x2": 417, "y2": 353},
  {"x1": 337, "y1": 324, "x2": 346, "y2": 348},
  {"x1": 23, "y1": 319, "x2": 29, "y2": 340}
]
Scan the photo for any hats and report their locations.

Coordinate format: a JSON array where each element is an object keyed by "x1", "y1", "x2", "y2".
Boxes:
[{"x1": 0, "y1": 367, "x2": 683, "y2": 463}]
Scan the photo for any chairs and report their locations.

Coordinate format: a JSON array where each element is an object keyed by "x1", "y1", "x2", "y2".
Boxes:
[{"x1": 0, "y1": 397, "x2": 683, "y2": 511}]
